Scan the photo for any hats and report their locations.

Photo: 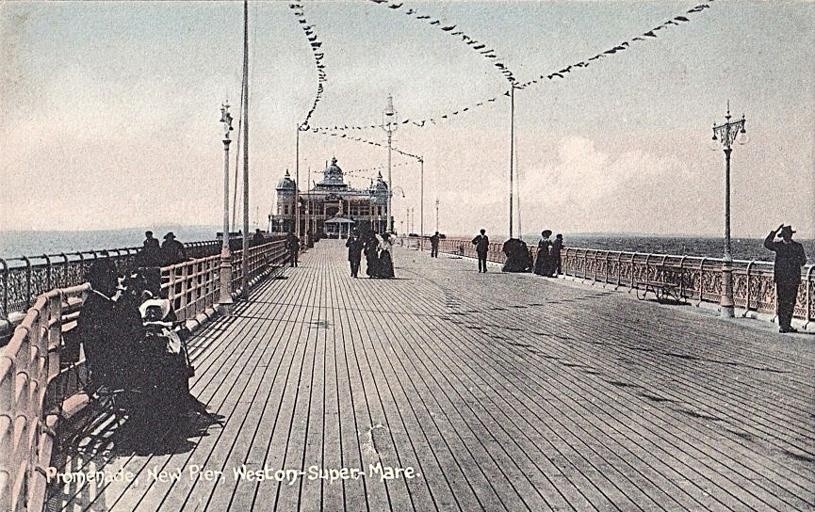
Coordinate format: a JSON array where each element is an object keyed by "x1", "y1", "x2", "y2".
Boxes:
[
  {"x1": 776, "y1": 225, "x2": 796, "y2": 238},
  {"x1": 163, "y1": 232, "x2": 176, "y2": 241},
  {"x1": 83, "y1": 258, "x2": 124, "y2": 283}
]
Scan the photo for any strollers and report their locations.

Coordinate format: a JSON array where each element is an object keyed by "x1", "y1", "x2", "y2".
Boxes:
[{"x1": 377, "y1": 247, "x2": 395, "y2": 280}]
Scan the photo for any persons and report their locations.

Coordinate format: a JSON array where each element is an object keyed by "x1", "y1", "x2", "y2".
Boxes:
[
  {"x1": 472, "y1": 229, "x2": 489, "y2": 273},
  {"x1": 346, "y1": 230, "x2": 394, "y2": 279},
  {"x1": 502, "y1": 230, "x2": 565, "y2": 278},
  {"x1": 764, "y1": 224, "x2": 806, "y2": 333},
  {"x1": 78, "y1": 232, "x2": 213, "y2": 456},
  {"x1": 253, "y1": 229, "x2": 264, "y2": 245},
  {"x1": 430, "y1": 232, "x2": 439, "y2": 258},
  {"x1": 287, "y1": 233, "x2": 300, "y2": 267}
]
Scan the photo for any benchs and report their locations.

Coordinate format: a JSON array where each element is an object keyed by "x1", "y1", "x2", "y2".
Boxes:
[
  {"x1": 262, "y1": 249, "x2": 287, "y2": 281},
  {"x1": 636, "y1": 265, "x2": 689, "y2": 307},
  {"x1": 50, "y1": 315, "x2": 198, "y2": 457}
]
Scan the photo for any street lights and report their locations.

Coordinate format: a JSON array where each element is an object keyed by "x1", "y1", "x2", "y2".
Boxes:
[
  {"x1": 709, "y1": 98, "x2": 748, "y2": 318},
  {"x1": 392, "y1": 183, "x2": 407, "y2": 199},
  {"x1": 434, "y1": 195, "x2": 441, "y2": 234},
  {"x1": 214, "y1": 94, "x2": 239, "y2": 306},
  {"x1": 383, "y1": 93, "x2": 397, "y2": 236}
]
